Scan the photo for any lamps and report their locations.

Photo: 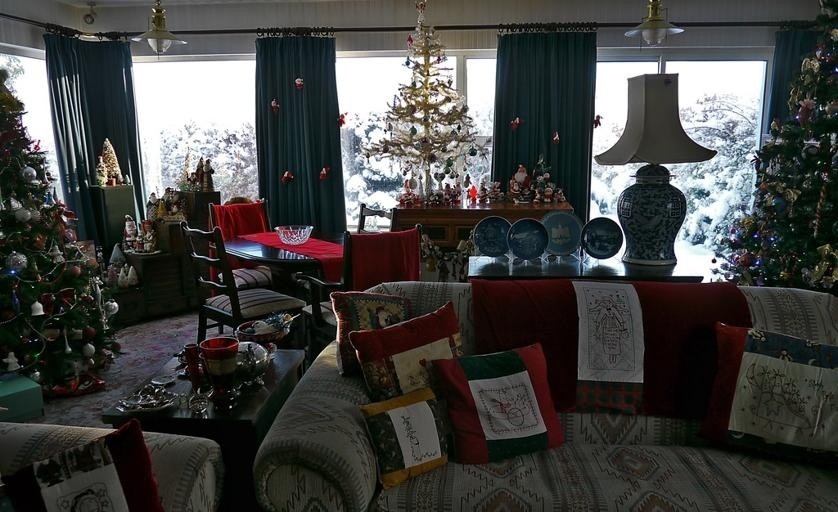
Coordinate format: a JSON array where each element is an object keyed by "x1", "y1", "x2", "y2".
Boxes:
[
  {"x1": 595, "y1": 74, "x2": 717, "y2": 267},
  {"x1": 131, "y1": 0, "x2": 188, "y2": 55},
  {"x1": 624, "y1": 0, "x2": 685, "y2": 47}
]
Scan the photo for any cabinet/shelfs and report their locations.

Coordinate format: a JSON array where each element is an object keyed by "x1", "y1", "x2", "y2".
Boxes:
[{"x1": 396, "y1": 201, "x2": 575, "y2": 247}]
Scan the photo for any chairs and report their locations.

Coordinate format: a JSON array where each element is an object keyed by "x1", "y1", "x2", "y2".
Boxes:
[
  {"x1": 180, "y1": 198, "x2": 422, "y2": 349},
  {"x1": 0, "y1": 422, "x2": 225, "y2": 512}
]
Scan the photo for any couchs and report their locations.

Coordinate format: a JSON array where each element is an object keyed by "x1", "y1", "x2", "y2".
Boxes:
[{"x1": 254, "y1": 280, "x2": 838, "y2": 512}]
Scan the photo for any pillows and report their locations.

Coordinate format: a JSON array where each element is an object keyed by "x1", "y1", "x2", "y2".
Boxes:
[
  {"x1": 696, "y1": 321, "x2": 838, "y2": 472},
  {"x1": 15, "y1": 418, "x2": 165, "y2": 512}
]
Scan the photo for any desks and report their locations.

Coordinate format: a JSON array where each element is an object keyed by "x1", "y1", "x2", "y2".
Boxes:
[{"x1": 467, "y1": 255, "x2": 703, "y2": 284}]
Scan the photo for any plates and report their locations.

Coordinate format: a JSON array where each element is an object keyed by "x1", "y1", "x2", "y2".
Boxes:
[
  {"x1": 115, "y1": 392, "x2": 178, "y2": 414},
  {"x1": 507, "y1": 218, "x2": 548, "y2": 259},
  {"x1": 471, "y1": 216, "x2": 512, "y2": 257},
  {"x1": 238, "y1": 341, "x2": 268, "y2": 366},
  {"x1": 539, "y1": 211, "x2": 582, "y2": 257},
  {"x1": 580, "y1": 216, "x2": 624, "y2": 259}
]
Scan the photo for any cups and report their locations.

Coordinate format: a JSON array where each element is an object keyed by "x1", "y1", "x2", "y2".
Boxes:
[
  {"x1": 108, "y1": 178, "x2": 116, "y2": 186},
  {"x1": 185, "y1": 343, "x2": 199, "y2": 361}
]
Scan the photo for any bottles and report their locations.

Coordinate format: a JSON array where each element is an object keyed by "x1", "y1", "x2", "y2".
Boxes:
[
  {"x1": 179, "y1": 391, "x2": 190, "y2": 409},
  {"x1": 95, "y1": 156, "x2": 107, "y2": 187},
  {"x1": 364, "y1": 214, "x2": 382, "y2": 233}
]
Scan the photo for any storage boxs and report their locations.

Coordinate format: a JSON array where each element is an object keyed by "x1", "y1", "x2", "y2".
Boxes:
[{"x1": 0, "y1": 375, "x2": 45, "y2": 422}]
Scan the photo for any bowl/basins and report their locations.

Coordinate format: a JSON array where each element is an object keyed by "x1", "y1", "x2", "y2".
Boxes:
[{"x1": 272, "y1": 222, "x2": 315, "y2": 247}]
